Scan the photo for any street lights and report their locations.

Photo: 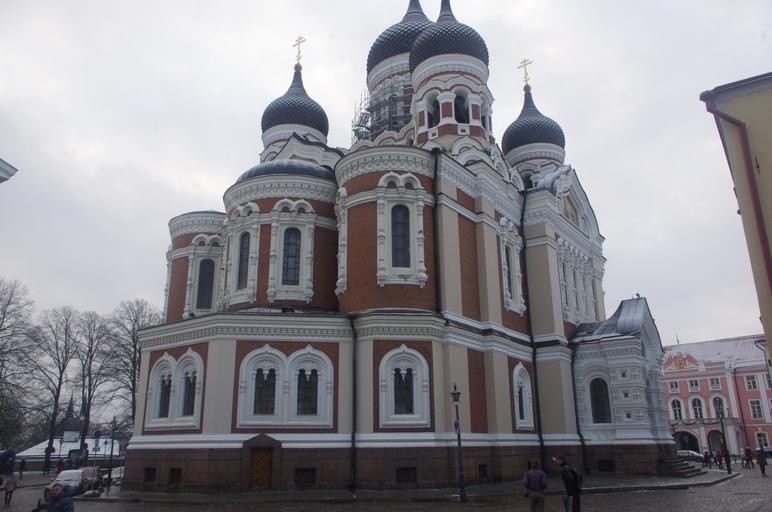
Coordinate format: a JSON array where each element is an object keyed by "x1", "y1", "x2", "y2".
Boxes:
[
  {"x1": 450, "y1": 383, "x2": 466, "y2": 504},
  {"x1": 712, "y1": 396, "x2": 733, "y2": 475},
  {"x1": 107, "y1": 416, "x2": 117, "y2": 489}
]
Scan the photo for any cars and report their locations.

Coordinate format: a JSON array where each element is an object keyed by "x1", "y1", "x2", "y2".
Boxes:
[
  {"x1": 44, "y1": 466, "x2": 123, "y2": 499},
  {"x1": 677, "y1": 449, "x2": 705, "y2": 463}
]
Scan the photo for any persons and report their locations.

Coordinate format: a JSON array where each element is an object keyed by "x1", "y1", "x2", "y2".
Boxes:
[
  {"x1": 702, "y1": 446, "x2": 767, "y2": 475},
  {"x1": 46, "y1": 484, "x2": 74, "y2": 512},
  {"x1": 2, "y1": 470, "x2": 18, "y2": 507},
  {"x1": 523, "y1": 458, "x2": 548, "y2": 512},
  {"x1": 18, "y1": 459, "x2": 26, "y2": 478},
  {"x1": 551, "y1": 452, "x2": 581, "y2": 511}
]
